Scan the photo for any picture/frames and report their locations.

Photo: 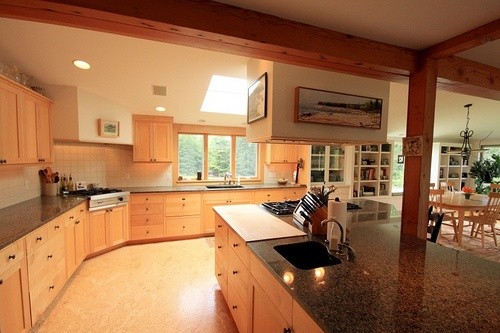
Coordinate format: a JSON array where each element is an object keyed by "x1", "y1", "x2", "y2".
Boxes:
[
  {"x1": 246, "y1": 72, "x2": 267, "y2": 123},
  {"x1": 294, "y1": 86, "x2": 383, "y2": 130},
  {"x1": 401, "y1": 135, "x2": 423, "y2": 158},
  {"x1": 98, "y1": 119, "x2": 121, "y2": 138}
]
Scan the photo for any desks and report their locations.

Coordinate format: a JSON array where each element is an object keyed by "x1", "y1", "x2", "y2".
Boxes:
[{"x1": 430, "y1": 192, "x2": 500, "y2": 247}]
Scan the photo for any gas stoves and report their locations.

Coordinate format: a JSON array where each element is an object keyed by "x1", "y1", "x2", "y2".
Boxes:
[
  {"x1": 261, "y1": 199, "x2": 362, "y2": 215},
  {"x1": 70, "y1": 189, "x2": 130, "y2": 211}
]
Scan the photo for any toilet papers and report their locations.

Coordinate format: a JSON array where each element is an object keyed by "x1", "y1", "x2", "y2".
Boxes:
[{"x1": 326, "y1": 199, "x2": 347, "y2": 244}]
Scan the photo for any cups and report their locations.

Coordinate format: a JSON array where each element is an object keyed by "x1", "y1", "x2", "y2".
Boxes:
[{"x1": 45, "y1": 182, "x2": 58, "y2": 196}]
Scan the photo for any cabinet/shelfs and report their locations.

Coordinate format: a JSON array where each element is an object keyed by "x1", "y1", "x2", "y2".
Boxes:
[
  {"x1": 0, "y1": 74, "x2": 56, "y2": 167},
  {"x1": 132, "y1": 114, "x2": 173, "y2": 163},
  {"x1": 0, "y1": 189, "x2": 323, "y2": 333},
  {"x1": 308, "y1": 142, "x2": 394, "y2": 196},
  {"x1": 429, "y1": 141, "x2": 473, "y2": 192}
]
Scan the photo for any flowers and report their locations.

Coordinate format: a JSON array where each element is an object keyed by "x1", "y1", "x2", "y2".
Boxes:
[{"x1": 462, "y1": 186, "x2": 474, "y2": 194}]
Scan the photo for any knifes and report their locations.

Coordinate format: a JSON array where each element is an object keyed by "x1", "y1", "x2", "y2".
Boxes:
[{"x1": 299, "y1": 192, "x2": 324, "y2": 225}]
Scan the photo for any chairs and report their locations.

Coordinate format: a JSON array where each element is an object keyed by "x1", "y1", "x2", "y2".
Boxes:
[{"x1": 428, "y1": 181, "x2": 500, "y2": 247}]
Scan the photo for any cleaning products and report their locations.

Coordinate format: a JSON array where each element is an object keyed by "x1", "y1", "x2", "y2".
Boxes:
[{"x1": 329, "y1": 221, "x2": 338, "y2": 250}]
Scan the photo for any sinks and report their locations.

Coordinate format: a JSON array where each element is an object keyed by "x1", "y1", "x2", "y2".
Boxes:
[
  {"x1": 205, "y1": 186, "x2": 245, "y2": 189},
  {"x1": 274, "y1": 240, "x2": 344, "y2": 272}
]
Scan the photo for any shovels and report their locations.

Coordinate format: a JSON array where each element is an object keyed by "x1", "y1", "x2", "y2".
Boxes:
[{"x1": 39, "y1": 167, "x2": 52, "y2": 183}]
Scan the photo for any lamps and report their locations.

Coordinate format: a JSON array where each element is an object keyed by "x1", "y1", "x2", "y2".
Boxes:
[{"x1": 460, "y1": 103, "x2": 473, "y2": 159}]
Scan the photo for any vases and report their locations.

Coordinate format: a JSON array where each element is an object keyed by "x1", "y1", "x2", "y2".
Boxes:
[{"x1": 464, "y1": 194, "x2": 471, "y2": 199}]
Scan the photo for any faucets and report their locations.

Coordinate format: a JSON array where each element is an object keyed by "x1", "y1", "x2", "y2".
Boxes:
[
  {"x1": 224, "y1": 172, "x2": 230, "y2": 185},
  {"x1": 320, "y1": 217, "x2": 348, "y2": 255}
]
